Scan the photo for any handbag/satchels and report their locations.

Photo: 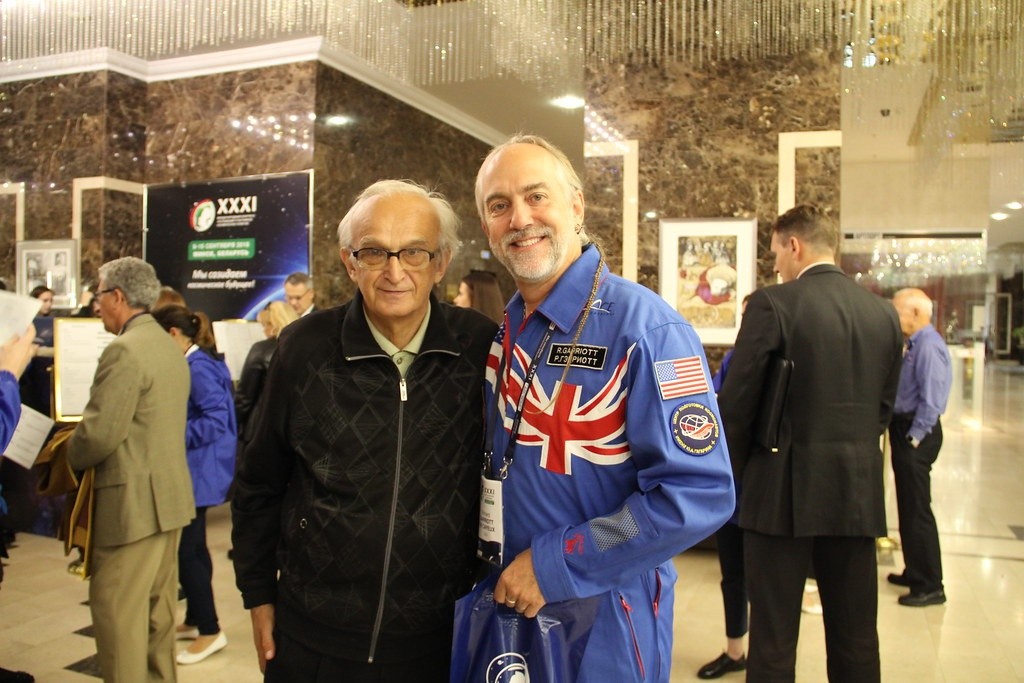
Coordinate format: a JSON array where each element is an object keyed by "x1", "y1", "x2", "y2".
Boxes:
[
  {"x1": 754, "y1": 357, "x2": 794, "y2": 451},
  {"x1": 452, "y1": 576, "x2": 570, "y2": 680}
]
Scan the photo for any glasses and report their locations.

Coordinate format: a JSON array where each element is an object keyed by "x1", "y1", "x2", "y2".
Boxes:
[
  {"x1": 284, "y1": 289, "x2": 310, "y2": 301},
  {"x1": 348, "y1": 248, "x2": 441, "y2": 271},
  {"x1": 95, "y1": 288, "x2": 117, "y2": 295}
]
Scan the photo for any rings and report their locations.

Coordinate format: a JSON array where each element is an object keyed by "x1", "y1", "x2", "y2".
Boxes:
[{"x1": 506, "y1": 596, "x2": 515, "y2": 603}]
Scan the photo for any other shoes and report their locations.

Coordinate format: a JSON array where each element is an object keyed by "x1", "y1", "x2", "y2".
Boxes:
[
  {"x1": 176, "y1": 625, "x2": 199, "y2": 641},
  {"x1": 175, "y1": 631, "x2": 228, "y2": 664}
]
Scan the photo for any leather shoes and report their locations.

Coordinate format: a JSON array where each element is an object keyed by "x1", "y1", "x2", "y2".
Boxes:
[
  {"x1": 888, "y1": 573, "x2": 922, "y2": 588},
  {"x1": 898, "y1": 588, "x2": 947, "y2": 607},
  {"x1": 696, "y1": 651, "x2": 746, "y2": 678}
]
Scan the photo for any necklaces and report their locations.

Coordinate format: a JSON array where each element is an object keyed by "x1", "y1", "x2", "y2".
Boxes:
[{"x1": 525, "y1": 246, "x2": 605, "y2": 415}]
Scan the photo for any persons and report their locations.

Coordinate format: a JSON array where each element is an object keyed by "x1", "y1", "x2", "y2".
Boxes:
[
  {"x1": 236, "y1": 273, "x2": 318, "y2": 422},
  {"x1": 0, "y1": 256, "x2": 236, "y2": 682},
  {"x1": 699, "y1": 295, "x2": 752, "y2": 678},
  {"x1": 887, "y1": 288, "x2": 949, "y2": 607},
  {"x1": 717, "y1": 204, "x2": 904, "y2": 683},
  {"x1": 475, "y1": 135, "x2": 736, "y2": 683},
  {"x1": 230, "y1": 180, "x2": 500, "y2": 683},
  {"x1": 451, "y1": 273, "x2": 503, "y2": 325}
]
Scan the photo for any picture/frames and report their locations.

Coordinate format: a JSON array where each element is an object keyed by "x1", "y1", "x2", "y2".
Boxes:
[
  {"x1": 657, "y1": 216, "x2": 757, "y2": 349},
  {"x1": 17, "y1": 238, "x2": 81, "y2": 310}
]
